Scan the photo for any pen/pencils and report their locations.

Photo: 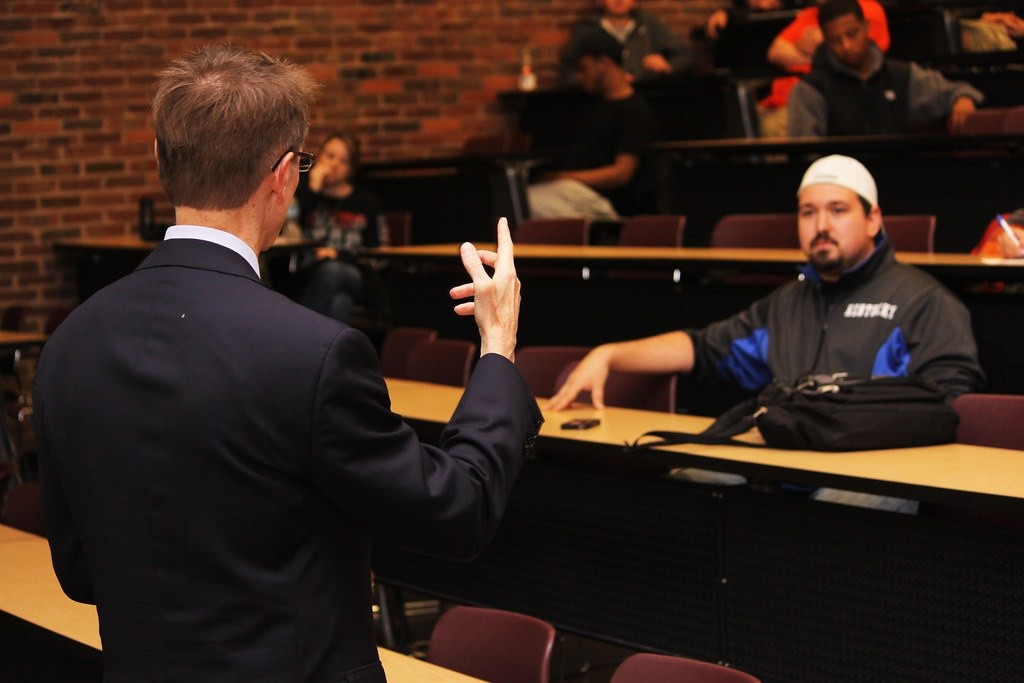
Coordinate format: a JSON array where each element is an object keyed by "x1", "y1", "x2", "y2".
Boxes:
[{"x1": 996, "y1": 215, "x2": 1019, "y2": 244}]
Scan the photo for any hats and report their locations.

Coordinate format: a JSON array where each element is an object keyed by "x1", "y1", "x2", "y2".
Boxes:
[{"x1": 798, "y1": 154, "x2": 878, "y2": 206}]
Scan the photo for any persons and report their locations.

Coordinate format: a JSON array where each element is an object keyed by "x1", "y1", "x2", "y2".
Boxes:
[
  {"x1": 19, "y1": 44, "x2": 546, "y2": 683},
  {"x1": 281, "y1": 123, "x2": 394, "y2": 346},
  {"x1": 542, "y1": 150, "x2": 985, "y2": 412},
  {"x1": 521, "y1": 0, "x2": 1024, "y2": 257}
]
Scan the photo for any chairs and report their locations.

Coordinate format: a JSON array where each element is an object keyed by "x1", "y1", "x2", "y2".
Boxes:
[
  {"x1": 883, "y1": 215, "x2": 936, "y2": 252},
  {"x1": 380, "y1": 328, "x2": 437, "y2": 380},
  {"x1": 946, "y1": 107, "x2": 1012, "y2": 134},
  {"x1": 512, "y1": 347, "x2": 678, "y2": 415},
  {"x1": 461, "y1": 133, "x2": 510, "y2": 153},
  {"x1": 610, "y1": 654, "x2": 760, "y2": 683},
  {"x1": 513, "y1": 218, "x2": 590, "y2": 245},
  {"x1": 618, "y1": 215, "x2": 686, "y2": 247},
  {"x1": 425, "y1": 605, "x2": 556, "y2": 683},
  {"x1": 404, "y1": 340, "x2": 476, "y2": 388},
  {"x1": 947, "y1": 394, "x2": 1024, "y2": 451},
  {"x1": 712, "y1": 213, "x2": 801, "y2": 249}
]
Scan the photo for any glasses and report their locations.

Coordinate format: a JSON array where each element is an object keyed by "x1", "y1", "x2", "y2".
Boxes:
[{"x1": 271, "y1": 146, "x2": 316, "y2": 173}]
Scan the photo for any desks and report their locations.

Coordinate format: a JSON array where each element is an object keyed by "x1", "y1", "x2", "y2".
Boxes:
[{"x1": 0, "y1": 48, "x2": 1024, "y2": 683}]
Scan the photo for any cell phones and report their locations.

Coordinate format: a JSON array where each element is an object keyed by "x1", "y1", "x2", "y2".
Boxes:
[{"x1": 562, "y1": 418, "x2": 600, "y2": 430}]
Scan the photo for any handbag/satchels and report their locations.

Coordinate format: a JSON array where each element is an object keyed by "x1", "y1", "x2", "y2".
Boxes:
[{"x1": 756, "y1": 372, "x2": 961, "y2": 450}]
